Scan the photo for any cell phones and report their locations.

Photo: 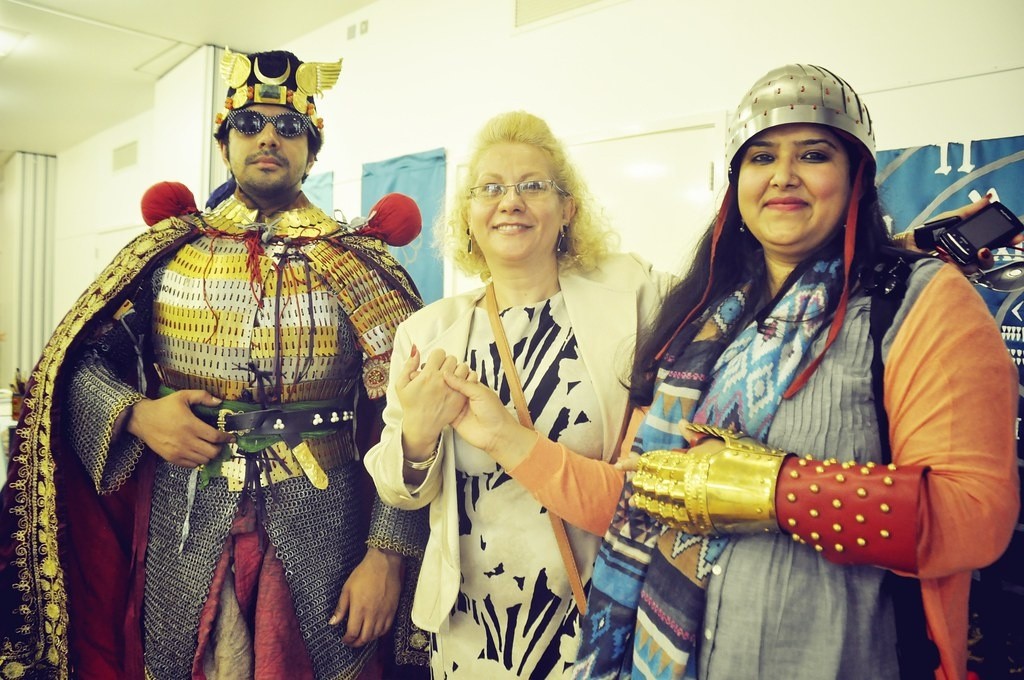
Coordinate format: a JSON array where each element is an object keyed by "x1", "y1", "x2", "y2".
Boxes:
[{"x1": 939, "y1": 201, "x2": 1024, "y2": 264}]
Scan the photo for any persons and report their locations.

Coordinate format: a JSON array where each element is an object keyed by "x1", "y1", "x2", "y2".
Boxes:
[
  {"x1": 362, "y1": 113, "x2": 1024, "y2": 680},
  {"x1": 410, "y1": 64, "x2": 1020, "y2": 680},
  {"x1": 4, "y1": 52, "x2": 430, "y2": 680}
]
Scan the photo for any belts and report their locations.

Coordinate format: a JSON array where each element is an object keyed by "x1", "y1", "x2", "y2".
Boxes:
[{"x1": 191, "y1": 404, "x2": 358, "y2": 492}]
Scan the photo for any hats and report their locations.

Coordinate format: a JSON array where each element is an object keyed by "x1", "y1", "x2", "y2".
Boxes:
[
  {"x1": 212, "y1": 46, "x2": 345, "y2": 163},
  {"x1": 728, "y1": 64, "x2": 879, "y2": 181}
]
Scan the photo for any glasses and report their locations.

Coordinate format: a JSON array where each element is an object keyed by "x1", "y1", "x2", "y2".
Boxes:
[
  {"x1": 466, "y1": 179, "x2": 570, "y2": 201},
  {"x1": 226, "y1": 110, "x2": 322, "y2": 140}
]
